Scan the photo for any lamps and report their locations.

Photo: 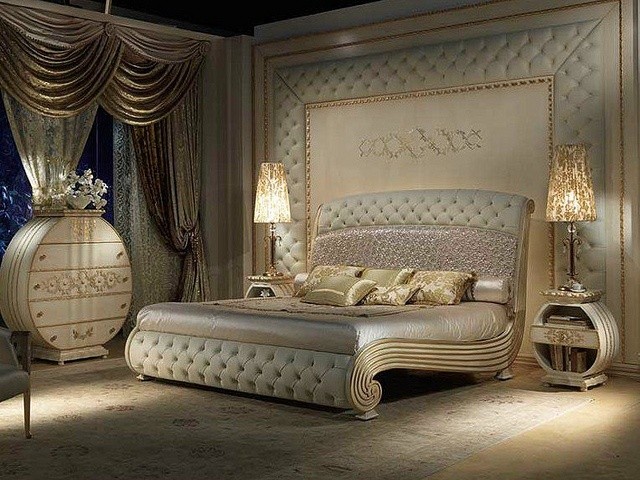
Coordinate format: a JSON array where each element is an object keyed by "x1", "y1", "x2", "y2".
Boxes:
[
  {"x1": 252, "y1": 162, "x2": 292, "y2": 277},
  {"x1": 544, "y1": 142, "x2": 597, "y2": 288}
]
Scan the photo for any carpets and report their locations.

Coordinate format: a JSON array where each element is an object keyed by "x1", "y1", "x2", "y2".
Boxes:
[{"x1": 0, "y1": 356, "x2": 593, "y2": 479}]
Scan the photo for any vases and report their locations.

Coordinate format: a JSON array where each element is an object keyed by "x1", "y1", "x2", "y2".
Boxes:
[{"x1": 68, "y1": 195, "x2": 91, "y2": 209}]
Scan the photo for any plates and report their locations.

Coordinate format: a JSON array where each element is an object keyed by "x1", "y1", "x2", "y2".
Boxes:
[{"x1": 571, "y1": 289, "x2": 586, "y2": 293}]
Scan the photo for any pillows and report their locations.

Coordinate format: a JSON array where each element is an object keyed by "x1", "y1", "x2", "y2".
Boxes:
[
  {"x1": 408, "y1": 270, "x2": 475, "y2": 305},
  {"x1": 361, "y1": 267, "x2": 414, "y2": 286},
  {"x1": 463, "y1": 278, "x2": 511, "y2": 304},
  {"x1": 363, "y1": 284, "x2": 420, "y2": 305},
  {"x1": 301, "y1": 276, "x2": 378, "y2": 306},
  {"x1": 293, "y1": 264, "x2": 366, "y2": 297}
]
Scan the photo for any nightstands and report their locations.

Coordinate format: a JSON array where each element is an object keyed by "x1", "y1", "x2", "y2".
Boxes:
[
  {"x1": 528, "y1": 289, "x2": 620, "y2": 393},
  {"x1": 245, "y1": 275, "x2": 295, "y2": 299}
]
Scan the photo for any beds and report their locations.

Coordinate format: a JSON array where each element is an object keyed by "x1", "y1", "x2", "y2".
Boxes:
[{"x1": 124, "y1": 189, "x2": 535, "y2": 420}]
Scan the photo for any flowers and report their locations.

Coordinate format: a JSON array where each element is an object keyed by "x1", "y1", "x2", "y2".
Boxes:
[{"x1": 67, "y1": 169, "x2": 108, "y2": 210}]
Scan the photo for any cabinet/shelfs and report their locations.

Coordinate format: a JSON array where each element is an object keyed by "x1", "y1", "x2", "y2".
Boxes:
[{"x1": 0, "y1": 209, "x2": 134, "y2": 365}]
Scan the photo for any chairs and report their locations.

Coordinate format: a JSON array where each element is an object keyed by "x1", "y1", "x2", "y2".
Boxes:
[{"x1": 0, "y1": 327, "x2": 32, "y2": 439}]
